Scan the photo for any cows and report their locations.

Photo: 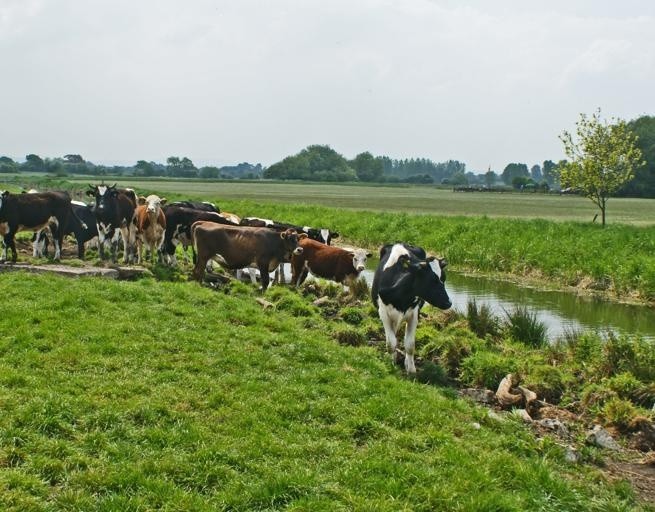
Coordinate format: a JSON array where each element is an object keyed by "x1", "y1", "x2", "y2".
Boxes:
[
  {"x1": 371, "y1": 241, "x2": 452, "y2": 377},
  {"x1": 0, "y1": 179, "x2": 372, "y2": 294}
]
